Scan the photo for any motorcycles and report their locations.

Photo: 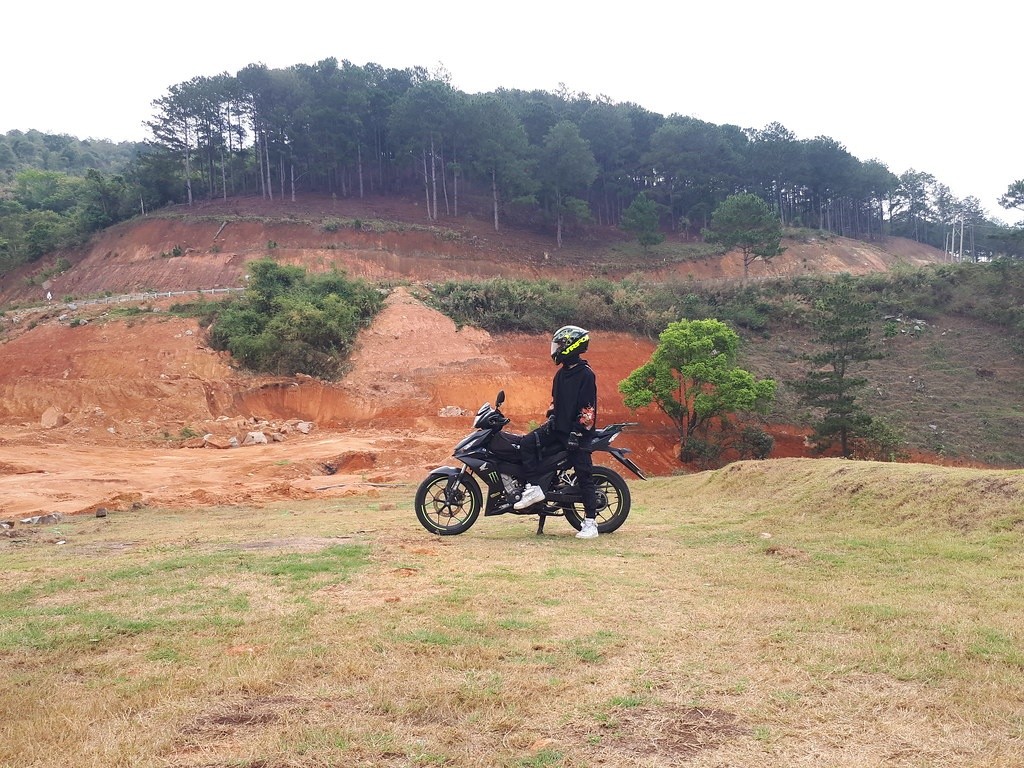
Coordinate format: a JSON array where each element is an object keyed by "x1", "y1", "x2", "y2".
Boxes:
[{"x1": 413, "y1": 389, "x2": 647, "y2": 537}]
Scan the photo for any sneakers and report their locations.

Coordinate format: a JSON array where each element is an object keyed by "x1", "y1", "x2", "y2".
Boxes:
[
  {"x1": 574, "y1": 517, "x2": 599, "y2": 540},
  {"x1": 513, "y1": 483, "x2": 545, "y2": 509}
]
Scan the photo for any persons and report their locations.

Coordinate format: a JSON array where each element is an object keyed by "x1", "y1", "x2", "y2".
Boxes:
[{"x1": 514, "y1": 326, "x2": 600, "y2": 540}]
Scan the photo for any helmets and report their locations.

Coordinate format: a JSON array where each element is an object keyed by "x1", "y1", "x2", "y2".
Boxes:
[{"x1": 551, "y1": 325, "x2": 590, "y2": 366}]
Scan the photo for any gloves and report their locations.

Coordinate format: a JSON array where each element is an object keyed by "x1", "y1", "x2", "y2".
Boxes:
[
  {"x1": 543, "y1": 409, "x2": 556, "y2": 436},
  {"x1": 565, "y1": 432, "x2": 583, "y2": 452}
]
